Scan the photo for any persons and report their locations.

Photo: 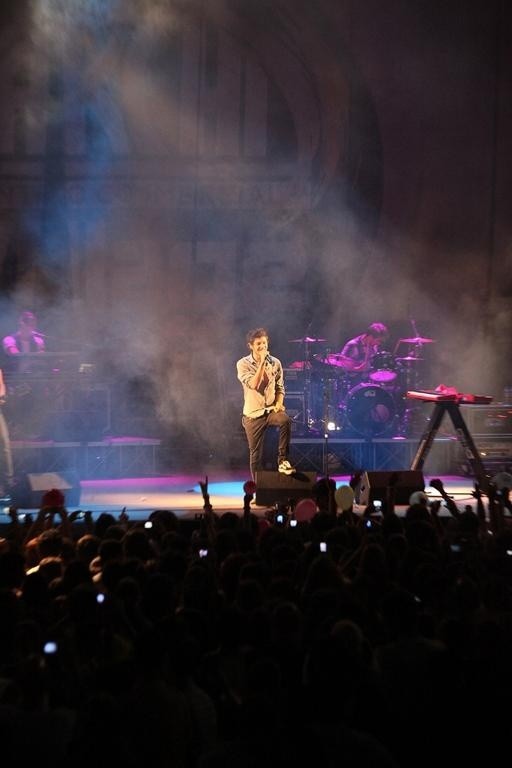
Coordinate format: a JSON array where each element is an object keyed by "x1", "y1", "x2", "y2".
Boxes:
[
  {"x1": 235, "y1": 325, "x2": 292, "y2": 504},
  {"x1": 1, "y1": 310, "x2": 47, "y2": 374},
  {"x1": 0, "y1": 365, "x2": 16, "y2": 497},
  {"x1": 341, "y1": 323, "x2": 391, "y2": 372},
  {"x1": 1, "y1": 472, "x2": 512, "y2": 768}
]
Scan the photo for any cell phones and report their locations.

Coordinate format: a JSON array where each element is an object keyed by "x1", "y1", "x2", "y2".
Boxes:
[{"x1": 319, "y1": 541, "x2": 327, "y2": 552}]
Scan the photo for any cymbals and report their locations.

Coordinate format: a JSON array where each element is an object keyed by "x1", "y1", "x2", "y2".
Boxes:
[
  {"x1": 395, "y1": 356, "x2": 426, "y2": 361},
  {"x1": 313, "y1": 354, "x2": 361, "y2": 368},
  {"x1": 401, "y1": 338, "x2": 436, "y2": 343},
  {"x1": 287, "y1": 337, "x2": 326, "y2": 342}
]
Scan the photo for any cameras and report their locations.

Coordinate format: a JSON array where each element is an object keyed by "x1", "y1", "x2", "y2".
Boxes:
[
  {"x1": 373, "y1": 499, "x2": 383, "y2": 509},
  {"x1": 289, "y1": 520, "x2": 298, "y2": 527},
  {"x1": 144, "y1": 521, "x2": 155, "y2": 530}
]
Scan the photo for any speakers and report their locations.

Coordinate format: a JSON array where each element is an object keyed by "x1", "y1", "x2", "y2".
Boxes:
[
  {"x1": 353, "y1": 472, "x2": 425, "y2": 505},
  {"x1": 10, "y1": 471, "x2": 82, "y2": 507},
  {"x1": 256, "y1": 471, "x2": 318, "y2": 505}
]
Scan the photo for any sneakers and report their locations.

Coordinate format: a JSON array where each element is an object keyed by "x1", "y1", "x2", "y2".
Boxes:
[{"x1": 277, "y1": 460, "x2": 296, "y2": 475}]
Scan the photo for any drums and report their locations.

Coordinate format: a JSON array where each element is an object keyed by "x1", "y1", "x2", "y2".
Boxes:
[
  {"x1": 342, "y1": 383, "x2": 396, "y2": 436},
  {"x1": 368, "y1": 352, "x2": 398, "y2": 382}
]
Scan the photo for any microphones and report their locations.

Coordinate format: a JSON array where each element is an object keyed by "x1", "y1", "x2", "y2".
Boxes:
[
  {"x1": 265, "y1": 355, "x2": 272, "y2": 364},
  {"x1": 31, "y1": 330, "x2": 50, "y2": 339}
]
